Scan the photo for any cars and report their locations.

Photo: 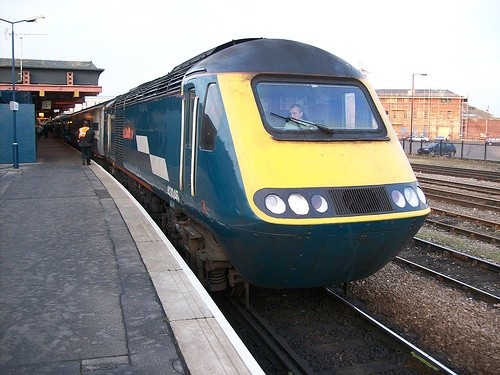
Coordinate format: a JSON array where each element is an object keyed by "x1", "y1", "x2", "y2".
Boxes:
[
  {"x1": 417, "y1": 140, "x2": 457, "y2": 157},
  {"x1": 407, "y1": 136, "x2": 429, "y2": 142}
]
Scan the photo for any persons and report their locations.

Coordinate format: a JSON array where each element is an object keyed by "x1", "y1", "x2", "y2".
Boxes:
[
  {"x1": 284, "y1": 104, "x2": 316, "y2": 129},
  {"x1": 75, "y1": 121, "x2": 95, "y2": 165},
  {"x1": 37, "y1": 124, "x2": 57, "y2": 139}
]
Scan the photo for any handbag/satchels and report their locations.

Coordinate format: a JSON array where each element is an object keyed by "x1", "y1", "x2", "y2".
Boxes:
[{"x1": 85, "y1": 129, "x2": 95, "y2": 142}]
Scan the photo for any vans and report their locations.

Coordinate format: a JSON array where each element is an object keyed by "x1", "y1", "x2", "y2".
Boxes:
[
  {"x1": 434, "y1": 135, "x2": 445, "y2": 141},
  {"x1": 484, "y1": 136, "x2": 500, "y2": 145}
]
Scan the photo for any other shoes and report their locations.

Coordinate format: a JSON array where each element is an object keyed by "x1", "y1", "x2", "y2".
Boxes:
[{"x1": 88, "y1": 162, "x2": 91, "y2": 165}]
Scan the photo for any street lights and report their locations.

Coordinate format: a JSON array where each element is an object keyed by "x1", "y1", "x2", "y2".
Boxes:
[
  {"x1": 0, "y1": 15, "x2": 46, "y2": 168},
  {"x1": 409, "y1": 73, "x2": 427, "y2": 153}
]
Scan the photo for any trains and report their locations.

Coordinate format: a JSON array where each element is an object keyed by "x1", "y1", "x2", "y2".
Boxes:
[{"x1": 46, "y1": 35, "x2": 432, "y2": 292}]
